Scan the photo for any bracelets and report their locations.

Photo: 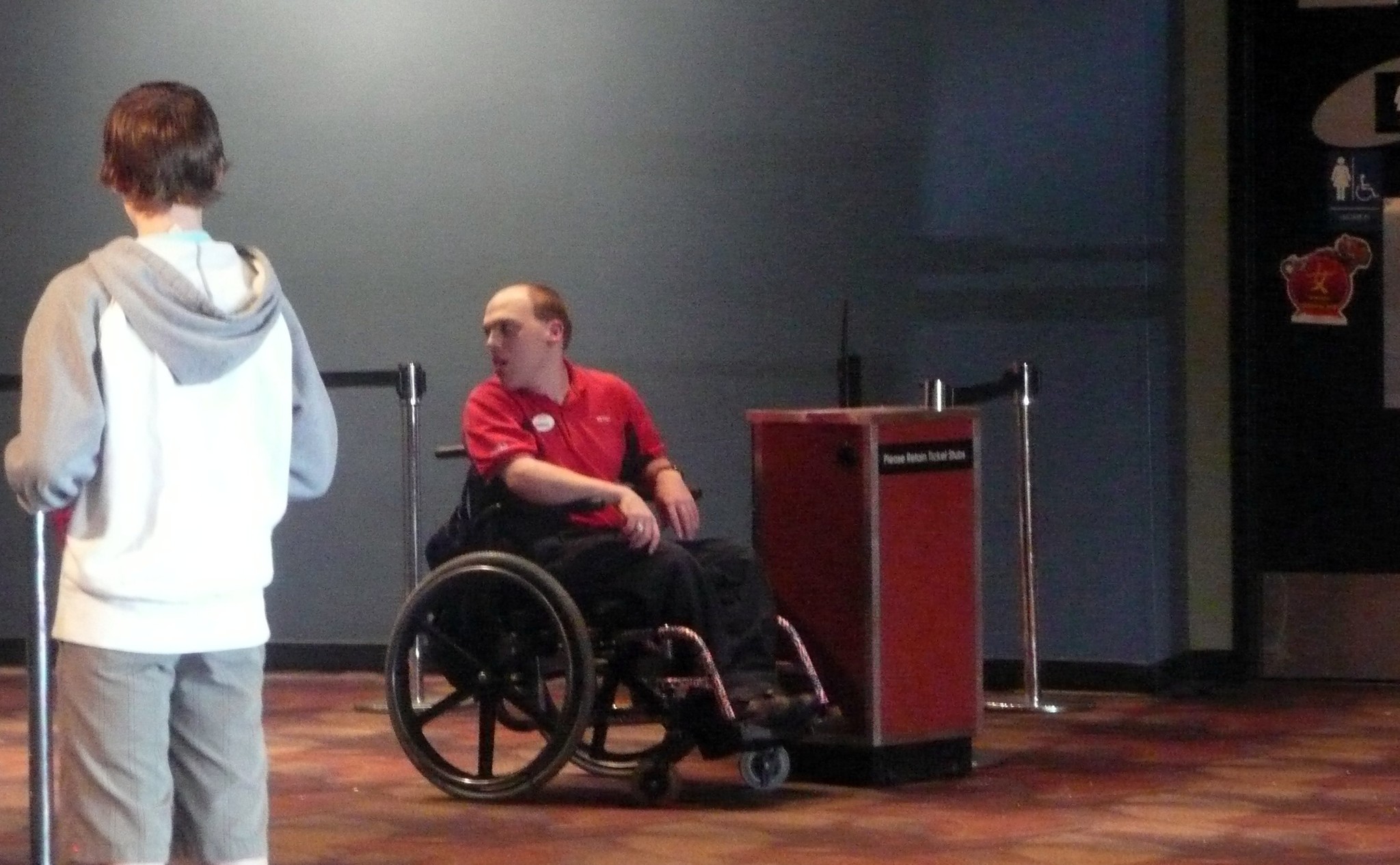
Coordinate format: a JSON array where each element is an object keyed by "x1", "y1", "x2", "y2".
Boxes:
[{"x1": 649, "y1": 462, "x2": 678, "y2": 487}]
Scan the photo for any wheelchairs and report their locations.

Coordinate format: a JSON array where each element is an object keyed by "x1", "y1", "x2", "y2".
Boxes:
[{"x1": 381, "y1": 438, "x2": 844, "y2": 810}]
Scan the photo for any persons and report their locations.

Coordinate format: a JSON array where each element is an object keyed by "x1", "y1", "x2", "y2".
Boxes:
[
  {"x1": 461, "y1": 284, "x2": 823, "y2": 754},
  {"x1": 4, "y1": 80, "x2": 339, "y2": 865}
]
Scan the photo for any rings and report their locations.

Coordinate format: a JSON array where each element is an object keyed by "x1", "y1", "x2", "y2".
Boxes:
[{"x1": 635, "y1": 524, "x2": 644, "y2": 532}]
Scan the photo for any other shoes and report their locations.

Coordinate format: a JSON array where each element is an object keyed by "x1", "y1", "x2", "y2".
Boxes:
[
  {"x1": 767, "y1": 691, "x2": 842, "y2": 732},
  {"x1": 701, "y1": 689, "x2": 787, "y2": 732}
]
